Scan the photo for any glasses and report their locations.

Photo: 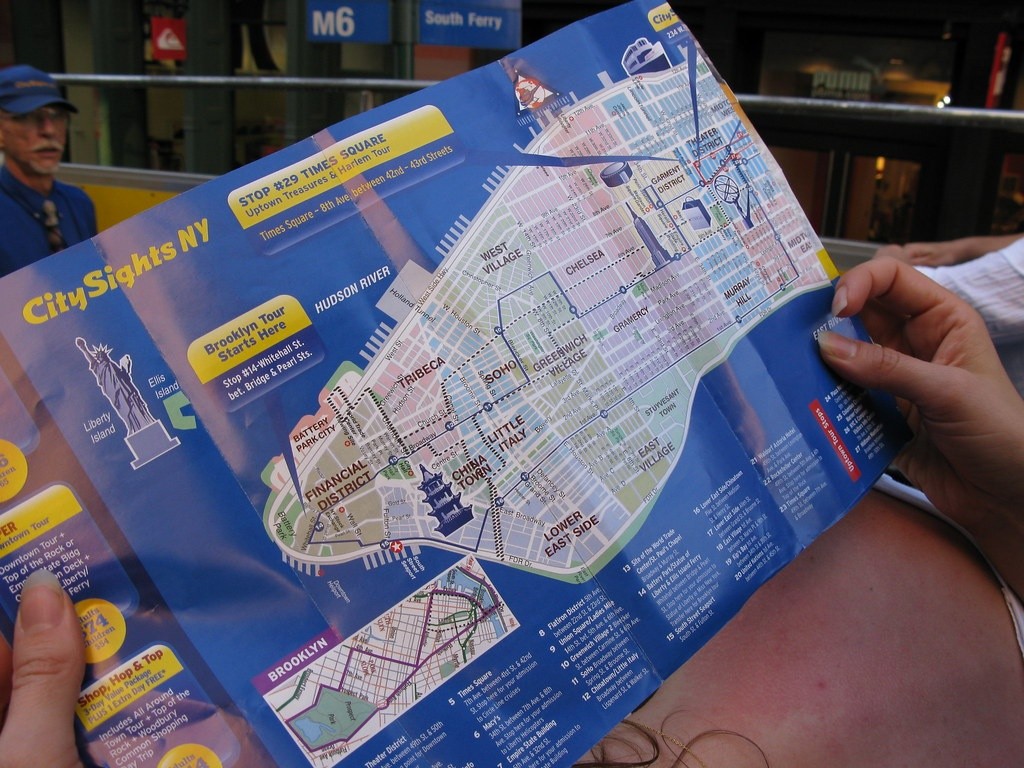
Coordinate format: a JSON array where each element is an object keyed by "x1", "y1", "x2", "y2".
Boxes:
[{"x1": 0, "y1": 110, "x2": 72, "y2": 134}]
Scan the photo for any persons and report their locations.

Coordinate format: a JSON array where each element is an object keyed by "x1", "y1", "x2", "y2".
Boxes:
[
  {"x1": 0, "y1": 63, "x2": 100, "y2": 277},
  {"x1": 0, "y1": 255, "x2": 1024, "y2": 768},
  {"x1": 156, "y1": 130, "x2": 185, "y2": 172},
  {"x1": 874, "y1": 233, "x2": 1024, "y2": 407}
]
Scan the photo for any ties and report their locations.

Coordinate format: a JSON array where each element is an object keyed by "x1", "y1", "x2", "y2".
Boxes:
[{"x1": 43, "y1": 200, "x2": 64, "y2": 255}]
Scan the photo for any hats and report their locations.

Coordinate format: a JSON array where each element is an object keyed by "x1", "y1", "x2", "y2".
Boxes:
[{"x1": 0, "y1": 64, "x2": 78, "y2": 115}]
